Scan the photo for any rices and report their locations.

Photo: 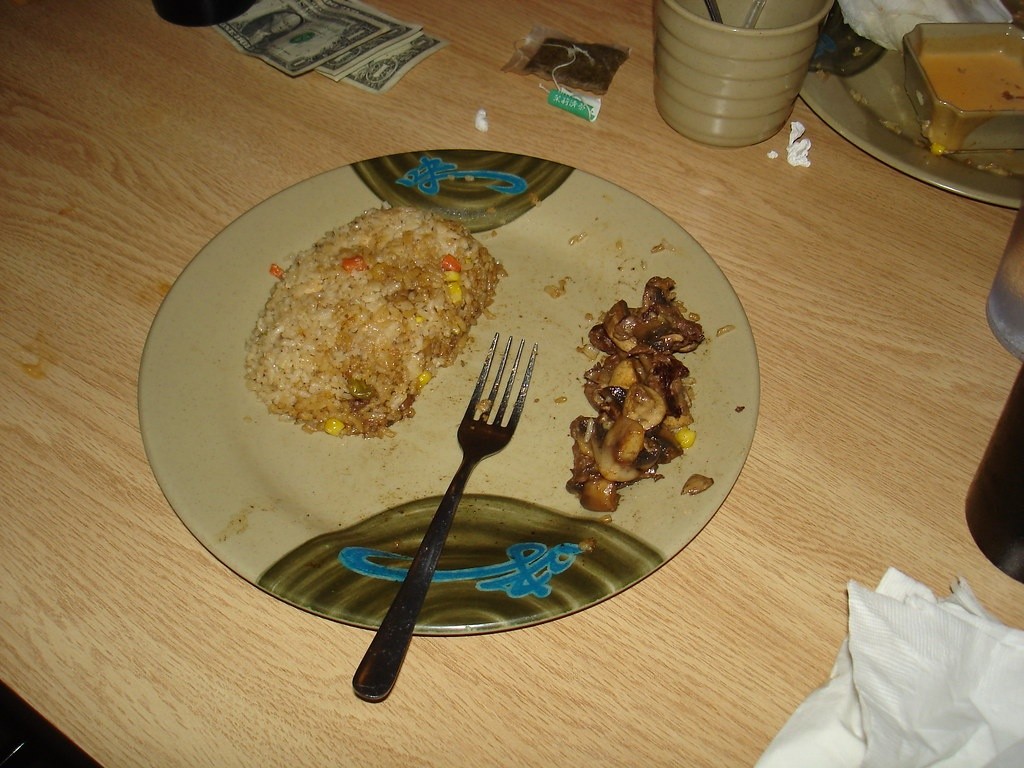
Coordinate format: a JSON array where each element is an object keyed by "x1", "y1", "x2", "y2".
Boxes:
[{"x1": 245, "y1": 199, "x2": 509, "y2": 438}]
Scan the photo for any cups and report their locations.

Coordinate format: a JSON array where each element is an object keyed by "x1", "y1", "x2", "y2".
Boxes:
[
  {"x1": 986, "y1": 195, "x2": 1024, "y2": 359},
  {"x1": 649, "y1": 1, "x2": 840, "y2": 148}
]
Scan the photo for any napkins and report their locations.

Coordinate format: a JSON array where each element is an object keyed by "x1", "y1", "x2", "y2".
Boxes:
[{"x1": 751, "y1": 562, "x2": 1024, "y2": 768}]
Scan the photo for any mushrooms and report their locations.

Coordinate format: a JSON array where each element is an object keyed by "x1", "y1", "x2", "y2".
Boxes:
[{"x1": 568, "y1": 277, "x2": 703, "y2": 512}]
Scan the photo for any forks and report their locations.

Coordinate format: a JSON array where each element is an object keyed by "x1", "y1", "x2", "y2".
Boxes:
[{"x1": 351, "y1": 334, "x2": 542, "y2": 703}]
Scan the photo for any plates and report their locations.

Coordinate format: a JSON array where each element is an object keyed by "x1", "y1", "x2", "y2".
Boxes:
[
  {"x1": 137, "y1": 150, "x2": 760, "y2": 635},
  {"x1": 797, "y1": 7, "x2": 1024, "y2": 211}
]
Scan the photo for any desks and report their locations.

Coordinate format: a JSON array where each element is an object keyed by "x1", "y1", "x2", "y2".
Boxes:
[{"x1": 0, "y1": 0, "x2": 1024, "y2": 768}]
